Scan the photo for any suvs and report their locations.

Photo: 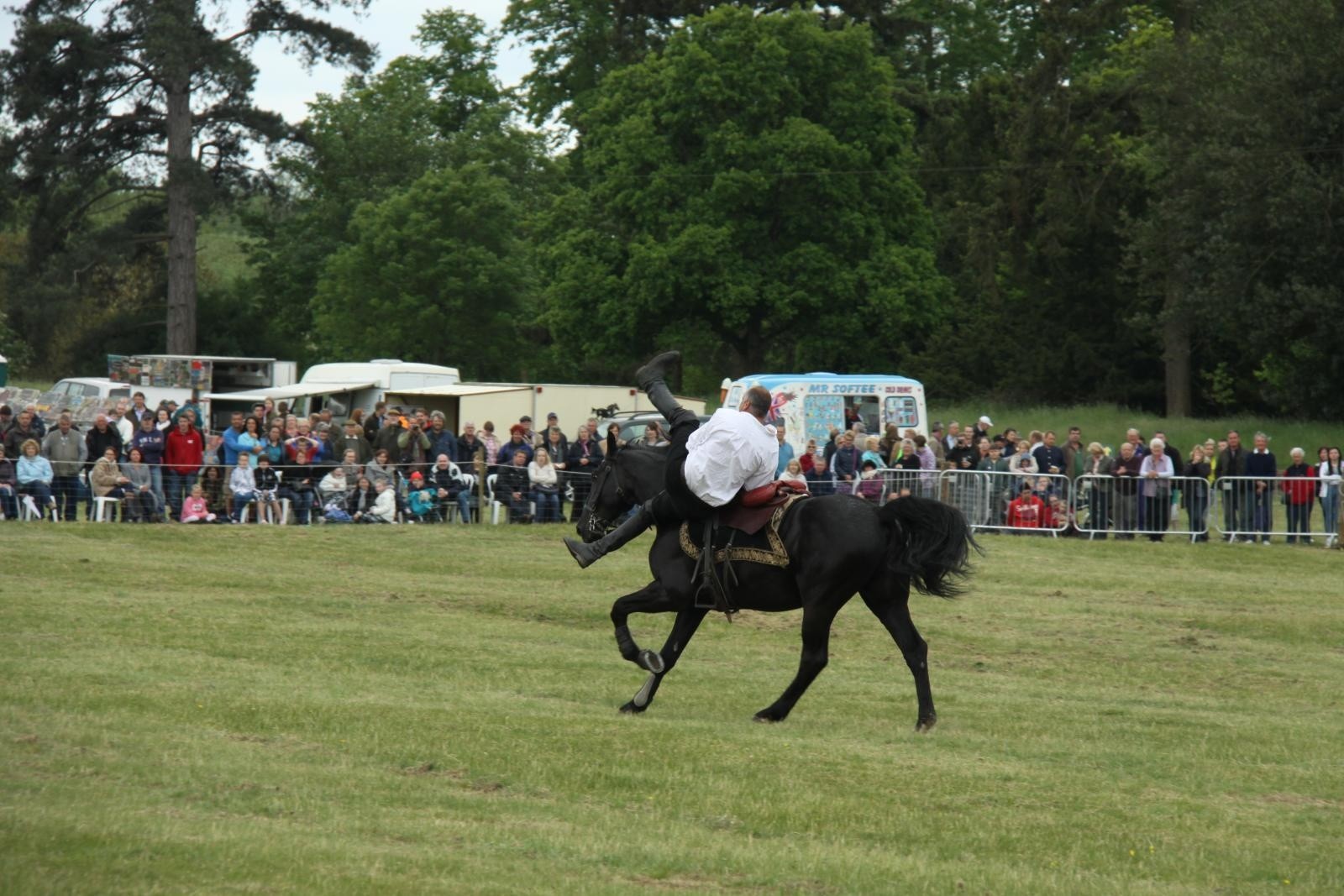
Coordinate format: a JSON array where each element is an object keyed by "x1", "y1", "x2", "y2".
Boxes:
[
  {"x1": 598, "y1": 408, "x2": 712, "y2": 448},
  {"x1": 46, "y1": 377, "x2": 132, "y2": 404}
]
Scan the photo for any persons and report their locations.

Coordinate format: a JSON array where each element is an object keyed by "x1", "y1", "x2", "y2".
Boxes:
[
  {"x1": 0, "y1": 392, "x2": 1344, "y2": 547},
  {"x1": 561, "y1": 350, "x2": 783, "y2": 565}
]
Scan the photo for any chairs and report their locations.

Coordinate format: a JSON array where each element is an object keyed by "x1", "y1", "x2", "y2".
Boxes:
[
  {"x1": 0, "y1": 458, "x2": 58, "y2": 523},
  {"x1": 852, "y1": 483, "x2": 887, "y2": 506},
  {"x1": 398, "y1": 471, "x2": 474, "y2": 525},
  {"x1": 89, "y1": 470, "x2": 126, "y2": 523},
  {"x1": 487, "y1": 474, "x2": 510, "y2": 524},
  {"x1": 240, "y1": 470, "x2": 349, "y2": 525}
]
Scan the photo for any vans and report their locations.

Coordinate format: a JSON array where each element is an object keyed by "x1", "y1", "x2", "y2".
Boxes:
[{"x1": 718, "y1": 371, "x2": 930, "y2": 470}]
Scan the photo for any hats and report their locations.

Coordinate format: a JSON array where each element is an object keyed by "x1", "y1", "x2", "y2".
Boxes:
[
  {"x1": 1020, "y1": 453, "x2": 1029, "y2": 460},
  {"x1": 980, "y1": 414, "x2": 994, "y2": 427},
  {"x1": 341, "y1": 420, "x2": 355, "y2": 426},
  {"x1": 409, "y1": 472, "x2": 422, "y2": 481},
  {"x1": 295, "y1": 417, "x2": 309, "y2": 426},
  {"x1": 548, "y1": 412, "x2": 557, "y2": 420},
  {"x1": 168, "y1": 401, "x2": 177, "y2": 406},
  {"x1": 141, "y1": 411, "x2": 152, "y2": 420},
  {"x1": 387, "y1": 409, "x2": 399, "y2": 416}
]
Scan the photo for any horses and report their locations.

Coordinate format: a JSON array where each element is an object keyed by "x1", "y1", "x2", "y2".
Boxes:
[{"x1": 576, "y1": 431, "x2": 986, "y2": 735}]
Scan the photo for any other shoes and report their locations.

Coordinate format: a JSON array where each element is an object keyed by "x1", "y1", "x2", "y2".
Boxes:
[
  {"x1": 228, "y1": 514, "x2": 238, "y2": 522},
  {"x1": 47, "y1": 503, "x2": 57, "y2": 509}
]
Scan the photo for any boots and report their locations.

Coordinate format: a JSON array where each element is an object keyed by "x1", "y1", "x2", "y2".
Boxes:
[
  {"x1": 635, "y1": 350, "x2": 684, "y2": 423},
  {"x1": 562, "y1": 512, "x2": 649, "y2": 569}
]
